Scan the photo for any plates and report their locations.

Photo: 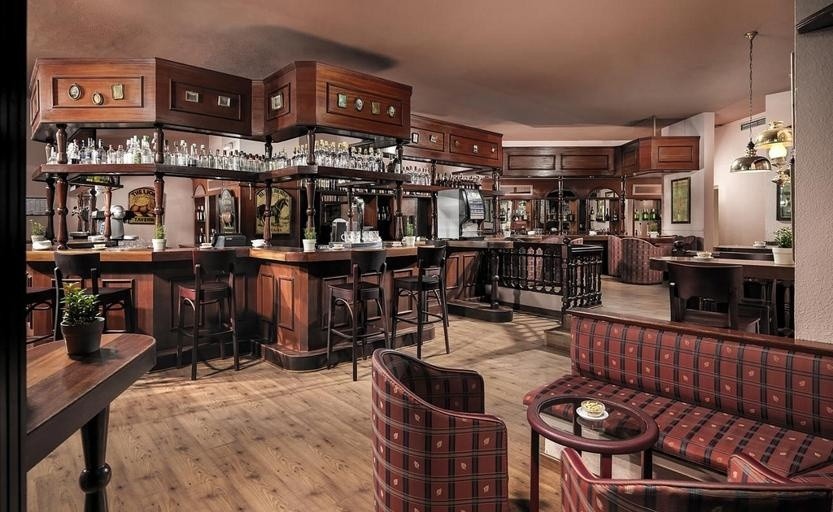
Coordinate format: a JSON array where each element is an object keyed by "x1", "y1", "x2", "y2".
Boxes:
[
  {"x1": 92, "y1": 247, "x2": 107, "y2": 250},
  {"x1": 694, "y1": 256, "x2": 714, "y2": 260},
  {"x1": 332, "y1": 247, "x2": 344, "y2": 250},
  {"x1": 577, "y1": 407, "x2": 609, "y2": 421},
  {"x1": 200, "y1": 246, "x2": 213, "y2": 249}
]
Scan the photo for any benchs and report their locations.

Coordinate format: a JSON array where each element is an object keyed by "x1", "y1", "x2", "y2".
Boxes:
[{"x1": 521, "y1": 306, "x2": 833, "y2": 484}]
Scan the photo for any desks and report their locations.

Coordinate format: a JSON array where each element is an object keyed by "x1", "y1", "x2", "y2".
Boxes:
[{"x1": 25, "y1": 333, "x2": 158, "y2": 512}]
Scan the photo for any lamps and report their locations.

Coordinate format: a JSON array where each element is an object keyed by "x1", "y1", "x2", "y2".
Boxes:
[
  {"x1": 729, "y1": 30, "x2": 771, "y2": 173},
  {"x1": 753, "y1": 121, "x2": 791, "y2": 209}
]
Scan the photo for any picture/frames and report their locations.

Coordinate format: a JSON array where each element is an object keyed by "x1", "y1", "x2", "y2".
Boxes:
[
  {"x1": 671, "y1": 177, "x2": 691, "y2": 224},
  {"x1": 776, "y1": 171, "x2": 791, "y2": 222},
  {"x1": 26, "y1": 197, "x2": 46, "y2": 216}
]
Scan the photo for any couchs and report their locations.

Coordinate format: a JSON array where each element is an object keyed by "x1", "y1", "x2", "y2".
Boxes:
[
  {"x1": 619, "y1": 237, "x2": 663, "y2": 284},
  {"x1": 607, "y1": 235, "x2": 622, "y2": 278},
  {"x1": 677, "y1": 236, "x2": 698, "y2": 252}
]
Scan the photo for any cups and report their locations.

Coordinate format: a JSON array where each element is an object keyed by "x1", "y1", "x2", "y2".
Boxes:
[{"x1": 341, "y1": 230, "x2": 379, "y2": 242}]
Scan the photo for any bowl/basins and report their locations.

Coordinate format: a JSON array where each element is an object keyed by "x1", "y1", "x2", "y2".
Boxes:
[
  {"x1": 333, "y1": 244, "x2": 343, "y2": 247},
  {"x1": 94, "y1": 244, "x2": 105, "y2": 248},
  {"x1": 696, "y1": 252, "x2": 712, "y2": 257},
  {"x1": 202, "y1": 243, "x2": 211, "y2": 246},
  {"x1": 581, "y1": 400, "x2": 605, "y2": 416}
]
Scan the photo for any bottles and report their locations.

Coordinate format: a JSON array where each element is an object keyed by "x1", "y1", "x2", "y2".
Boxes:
[
  {"x1": 162, "y1": 138, "x2": 266, "y2": 173},
  {"x1": 633, "y1": 207, "x2": 659, "y2": 221},
  {"x1": 313, "y1": 138, "x2": 400, "y2": 174},
  {"x1": 590, "y1": 207, "x2": 595, "y2": 221},
  {"x1": 435, "y1": 172, "x2": 483, "y2": 190},
  {"x1": 268, "y1": 148, "x2": 290, "y2": 171},
  {"x1": 401, "y1": 165, "x2": 433, "y2": 187},
  {"x1": 290, "y1": 143, "x2": 309, "y2": 167},
  {"x1": 47, "y1": 135, "x2": 155, "y2": 165}
]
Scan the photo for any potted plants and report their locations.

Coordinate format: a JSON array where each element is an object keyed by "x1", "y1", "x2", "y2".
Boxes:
[
  {"x1": 30, "y1": 220, "x2": 46, "y2": 242},
  {"x1": 60, "y1": 283, "x2": 105, "y2": 355},
  {"x1": 772, "y1": 227, "x2": 794, "y2": 264},
  {"x1": 301, "y1": 227, "x2": 317, "y2": 252},
  {"x1": 151, "y1": 221, "x2": 167, "y2": 250},
  {"x1": 405, "y1": 223, "x2": 415, "y2": 246}
]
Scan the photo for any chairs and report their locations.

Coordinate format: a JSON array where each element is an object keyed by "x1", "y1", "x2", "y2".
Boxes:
[
  {"x1": 667, "y1": 261, "x2": 760, "y2": 338},
  {"x1": 177, "y1": 249, "x2": 239, "y2": 382},
  {"x1": 326, "y1": 249, "x2": 390, "y2": 380},
  {"x1": 26, "y1": 286, "x2": 56, "y2": 348},
  {"x1": 53, "y1": 251, "x2": 132, "y2": 340},
  {"x1": 372, "y1": 346, "x2": 509, "y2": 512},
  {"x1": 392, "y1": 244, "x2": 451, "y2": 360},
  {"x1": 560, "y1": 448, "x2": 833, "y2": 512},
  {"x1": 718, "y1": 251, "x2": 778, "y2": 338}
]
